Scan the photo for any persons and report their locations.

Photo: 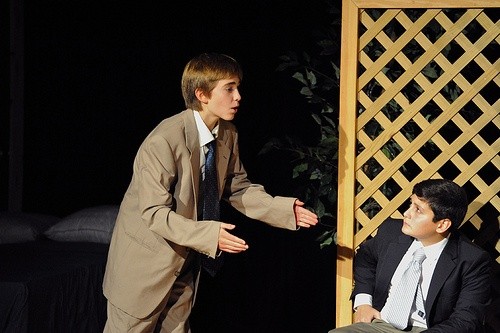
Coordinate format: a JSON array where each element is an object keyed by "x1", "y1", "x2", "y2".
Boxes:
[
  {"x1": 102, "y1": 53, "x2": 319, "y2": 333},
  {"x1": 328, "y1": 179, "x2": 491, "y2": 333}
]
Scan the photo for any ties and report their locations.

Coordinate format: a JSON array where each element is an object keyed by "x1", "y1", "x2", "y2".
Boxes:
[
  {"x1": 386, "y1": 248, "x2": 426, "y2": 331},
  {"x1": 202, "y1": 140, "x2": 220, "y2": 221}
]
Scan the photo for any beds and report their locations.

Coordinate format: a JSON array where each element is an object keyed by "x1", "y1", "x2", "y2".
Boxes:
[{"x1": 0, "y1": 242, "x2": 109, "y2": 333}]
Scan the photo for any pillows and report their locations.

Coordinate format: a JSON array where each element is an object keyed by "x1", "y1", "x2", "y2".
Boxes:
[
  {"x1": 0, "y1": 214, "x2": 62, "y2": 244},
  {"x1": 42, "y1": 206, "x2": 119, "y2": 244}
]
